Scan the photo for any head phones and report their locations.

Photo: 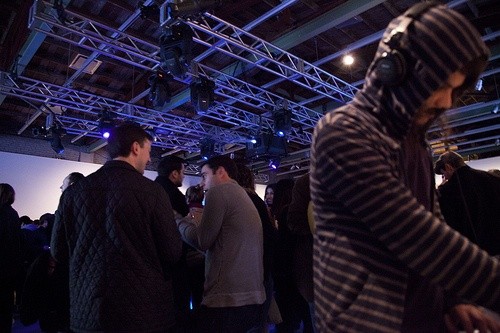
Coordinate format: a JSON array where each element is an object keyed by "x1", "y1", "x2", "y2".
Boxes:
[{"x1": 372, "y1": 0, "x2": 447, "y2": 87}]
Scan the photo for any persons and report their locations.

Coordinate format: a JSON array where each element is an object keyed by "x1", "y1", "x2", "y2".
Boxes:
[
  {"x1": 434, "y1": 150, "x2": 500, "y2": 256},
  {"x1": 309, "y1": 1, "x2": 500, "y2": 333},
  {"x1": 51, "y1": 124, "x2": 182, "y2": 333},
  {"x1": 0, "y1": 155, "x2": 317, "y2": 333}
]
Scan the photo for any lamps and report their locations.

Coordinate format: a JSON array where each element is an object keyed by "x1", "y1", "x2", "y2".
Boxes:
[
  {"x1": 200, "y1": 138, "x2": 215, "y2": 161},
  {"x1": 475, "y1": 79, "x2": 483, "y2": 92},
  {"x1": 100, "y1": 118, "x2": 114, "y2": 139},
  {"x1": 159, "y1": 22, "x2": 193, "y2": 78},
  {"x1": 149, "y1": 75, "x2": 171, "y2": 107},
  {"x1": 492, "y1": 103, "x2": 500, "y2": 114},
  {"x1": 49, "y1": 132, "x2": 65, "y2": 155},
  {"x1": 191, "y1": 78, "x2": 216, "y2": 115},
  {"x1": 269, "y1": 157, "x2": 281, "y2": 168},
  {"x1": 273, "y1": 110, "x2": 292, "y2": 137}
]
